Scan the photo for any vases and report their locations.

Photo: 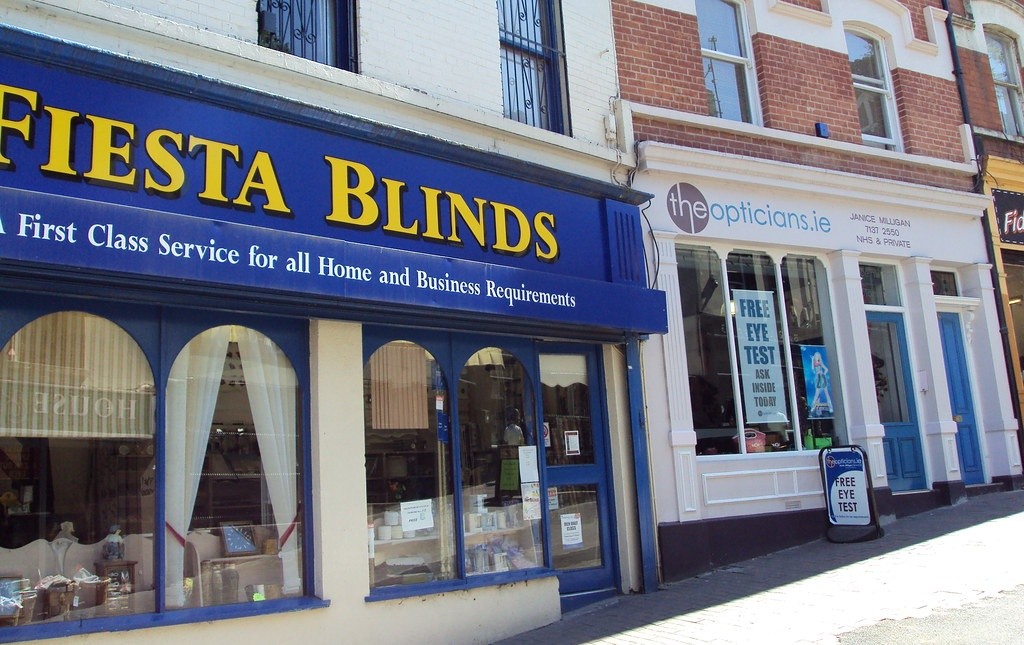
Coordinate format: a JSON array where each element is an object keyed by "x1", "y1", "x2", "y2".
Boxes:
[{"x1": 104, "y1": 534, "x2": 125, "y2": 560}]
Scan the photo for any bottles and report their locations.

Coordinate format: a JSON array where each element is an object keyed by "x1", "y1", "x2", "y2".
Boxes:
[{"x1": 201, "y1": 559, "x2": 239, "y2": 607}]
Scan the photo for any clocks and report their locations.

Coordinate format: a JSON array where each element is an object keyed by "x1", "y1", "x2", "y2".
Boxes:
[{"x1": 220, "y1": 519, "x2": 261, "y2": 557}]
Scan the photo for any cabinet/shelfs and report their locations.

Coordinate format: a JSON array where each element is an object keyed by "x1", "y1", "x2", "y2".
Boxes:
[
  {"x1": 100, "y1": 455, "x2": 157, "y2": 532},
  {"x1": 365, "y1": 448, "x2": 439, "y2": 506},
  {"x1": 185, "y1": 506, "x2": 535, "y2": 609},
  {"x1": 94, "y1": 561, "x2": 139, "y2": 614}
]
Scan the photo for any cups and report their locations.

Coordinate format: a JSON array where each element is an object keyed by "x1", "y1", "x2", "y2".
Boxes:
[{"x1": 377, "y1": 511, "x2": 416, "y2": 539}]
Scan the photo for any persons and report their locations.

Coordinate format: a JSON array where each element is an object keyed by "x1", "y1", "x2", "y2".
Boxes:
[
  {"x1": 503, "y1": 408, "x2": 524, "y2": 445},
  {"x1": 767, "y1": 396, "x2": 822, "y2": 446}
]
